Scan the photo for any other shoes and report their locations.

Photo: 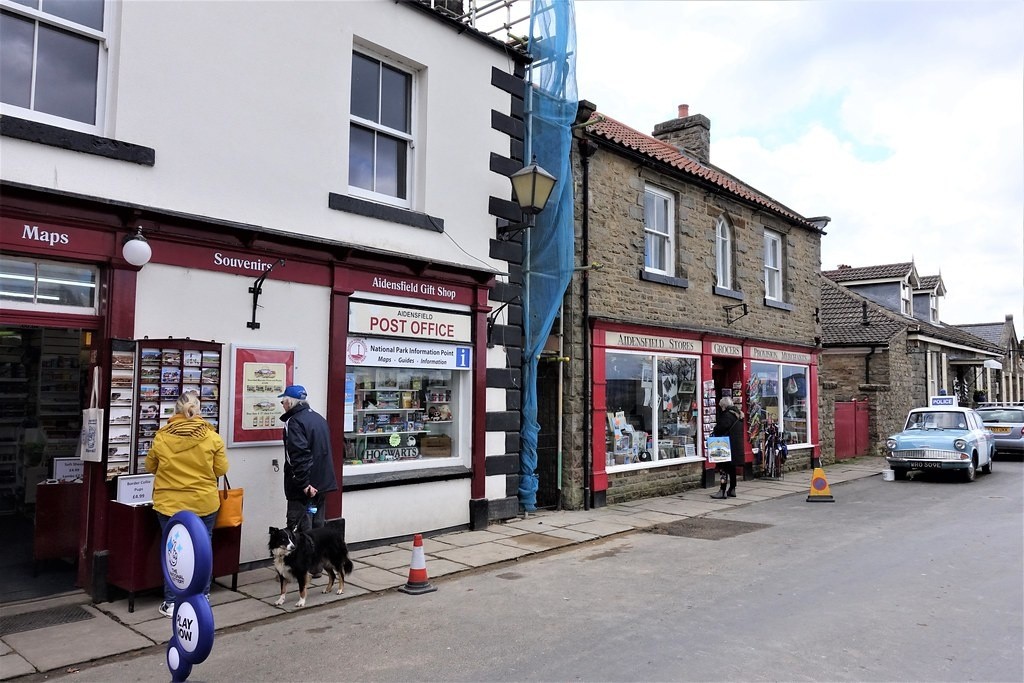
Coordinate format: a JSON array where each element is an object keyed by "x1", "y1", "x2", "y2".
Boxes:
[
  {"x1": 312, "y1": 571, "x2": 322, "y2": 577},
  {"x1": 727, "y1": 485, "x2": 736, "y2": 498},
  {"x1": 710, "y1": 489, "x2": 728, "y2": 498},
  {"x1": 158, "y1": 601, "x2": 174, "y2": 619}
]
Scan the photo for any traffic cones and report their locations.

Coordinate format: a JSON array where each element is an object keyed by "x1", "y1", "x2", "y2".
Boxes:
[{"x1": 398, "y1": 534, "x2": 438, "y2": 595}]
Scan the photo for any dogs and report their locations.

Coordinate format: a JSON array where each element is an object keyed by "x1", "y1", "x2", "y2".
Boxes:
[{"x1": 268, "y1": 517, "x2": 354, "y2": 607}]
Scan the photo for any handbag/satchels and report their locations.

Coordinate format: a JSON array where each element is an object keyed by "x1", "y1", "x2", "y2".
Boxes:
[
  {"x1": 705, "y1": 435, "x2": 732, "y2": 462},
  {"x1": 212, "y1": 471, "x2": 243, "y2": 529}
]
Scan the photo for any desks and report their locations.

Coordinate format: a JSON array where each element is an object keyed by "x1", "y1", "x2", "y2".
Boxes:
[
  {"x1": 106, "y1": 498, "x2": 242, "y2": 612},
  {"x1": 33, "y1": 478, "x2": 80, "y2": 578}
]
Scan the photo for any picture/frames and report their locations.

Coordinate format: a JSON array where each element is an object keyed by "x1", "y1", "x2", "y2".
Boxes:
[
  {"x1": 677, "y1": 399, "x2": 692, "y2": 412},
  {"x1": 678, "y1": 380, "x2": 695, "y2": 394}
]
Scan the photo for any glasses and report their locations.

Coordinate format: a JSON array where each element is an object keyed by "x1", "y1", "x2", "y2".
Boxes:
[{"x1": 281, "y1": 400, "x2": 286, "y2": 404}]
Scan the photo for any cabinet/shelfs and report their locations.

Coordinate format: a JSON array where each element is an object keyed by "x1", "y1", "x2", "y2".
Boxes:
[{"x1": 344, "y1": 365, "x2": 457, "y2": 464}]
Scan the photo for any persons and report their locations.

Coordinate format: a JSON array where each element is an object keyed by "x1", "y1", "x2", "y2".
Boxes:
[
  {"x1": 147, "y1": 405, "x2": 157, "y2": 418},
  {"x1": 1017, "y1": 400, "x2": 1024, "y2": 406},
  {"x1": 973, "y1": 390, "x2": 988, "y2": 406},
  {"x1": 277, "y1": 385, "x2": 339, "y2": 583},
  {"x1": 143, "y1": 441, "x2": 150, "y2": 448},
  {"x1": 708, "y1": 397, "x2": 745, "y2": 498},
  {"x1": 996, "y1": 394, "x2": 1003, "y2": 406},
  {"x1": 144, "y1": 391, "x2": 229, "y2": 620}
]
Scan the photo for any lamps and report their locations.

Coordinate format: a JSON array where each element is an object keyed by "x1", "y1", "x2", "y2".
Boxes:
[
  {"x1": 496, "y1": 153, "x2": 559, "y2": 242},
  {"x1": 121, "y1": 226, "x2": 152, "y2": 265}
]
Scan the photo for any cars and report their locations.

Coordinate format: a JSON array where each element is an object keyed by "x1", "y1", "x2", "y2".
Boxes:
[
  {"x1": 975, "y1": 406, "x2": 1024, "y2": 457},
  {"x1": 886, "y1": 406, "x2": 996, "y2": 481},
  {"x1": 783, "y1": 404, "x2": 808, "y2": 443}
]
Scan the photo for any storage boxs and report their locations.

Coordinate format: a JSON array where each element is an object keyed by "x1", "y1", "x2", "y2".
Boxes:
[{"x1": 410, "y1": 434, "x2": 451, "y2": 456}]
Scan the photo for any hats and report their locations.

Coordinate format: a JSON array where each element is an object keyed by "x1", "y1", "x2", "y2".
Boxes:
[{"x1": 278, "y1": 385, "x2": 307, "y2": 400}]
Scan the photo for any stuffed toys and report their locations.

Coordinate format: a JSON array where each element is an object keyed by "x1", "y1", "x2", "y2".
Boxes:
[
  {"x1": 431, "y1": 411, "x2": 441, "y2": 421},
  {"x1": 429, "y1": 407, "x2": 437, "y2": 420},
  {"x1": 406, "y1": 435, "x2": 416, "y2": 447},
  {"x1": 438, "y1": 405, "x2": 452, "y2": 420}
]
